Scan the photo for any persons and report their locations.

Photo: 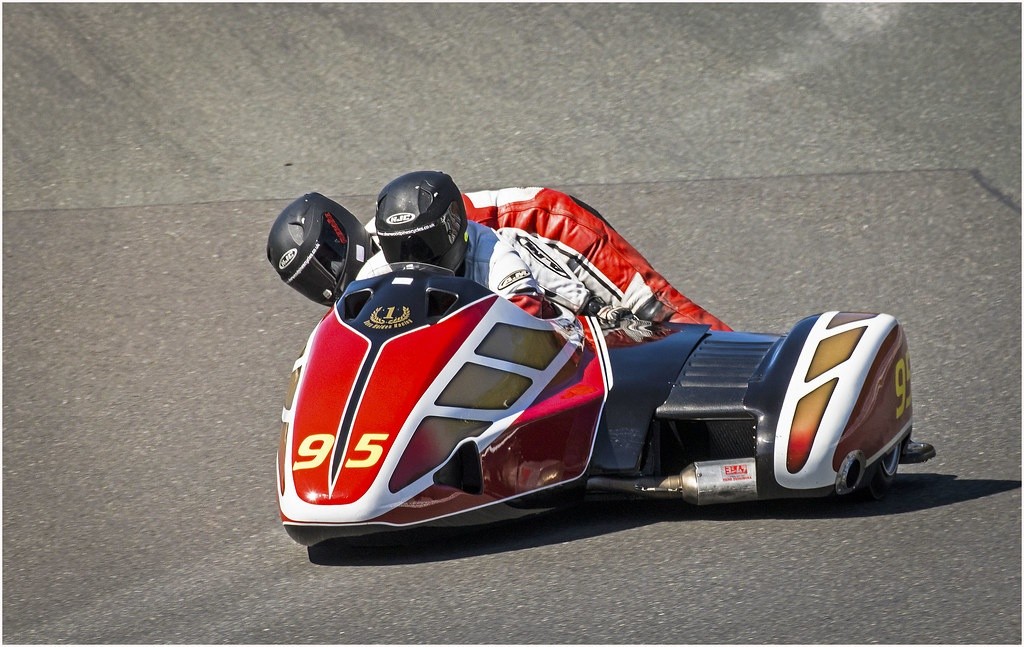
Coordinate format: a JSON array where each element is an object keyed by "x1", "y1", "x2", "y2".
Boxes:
[
  {"x1": 266, "y1": 187, "x2": 736, "y2": 343},
  {"x1": 356, "y1": 171, "x2": 545, "y2": 320}
]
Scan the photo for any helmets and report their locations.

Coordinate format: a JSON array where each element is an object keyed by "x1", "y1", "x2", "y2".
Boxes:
[
  {"x1": 373, "y1": 168, "x2": 469, "y2": 279},
  {"x1": 265, "y1": 190, "x2": 374, "y2": 310}
]
